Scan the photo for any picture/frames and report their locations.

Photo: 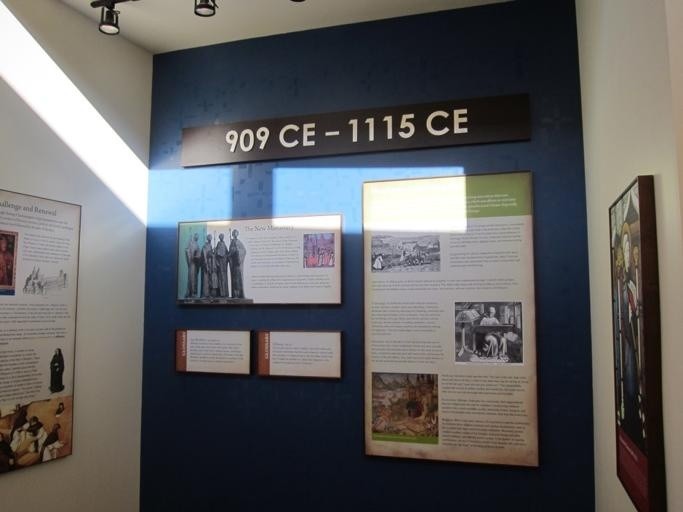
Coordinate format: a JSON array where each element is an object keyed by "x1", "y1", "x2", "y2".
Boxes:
[{"x1": 605, "y1": 166, "x2": 671, "y2": 512}]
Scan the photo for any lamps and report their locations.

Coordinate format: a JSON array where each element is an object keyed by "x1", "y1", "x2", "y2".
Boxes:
[{"x1": 85, "y1": 1, "x2": 233, "y2": 41}]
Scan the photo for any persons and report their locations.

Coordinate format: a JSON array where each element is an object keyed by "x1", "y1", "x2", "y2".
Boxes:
[
  {"x1": 214, "y1": 233, "x2": 228, "y2": 299},
  {"x1": 49, "y1": 346, "x2": 67, "y2": 394},
  {"x1": 480, "y1": 308, "x2": 512, "y2": 359},
  {"x1": 37, "y1": 422, "x2": 63, "y2": 463},
  {"x1": 55, "y1": 401, "x2": 69, "y2": 418},
  {"x1": 200, "y1": 234, "x2": 215, "y2": 297},
  {"x1": 10, "y1": 415, "x2": 45, "y2": 451},
  {"x1": 185, "y1": 233, "x2": 200, "y2": 297},
  {"x1": 617, "y1": 228, "x2": 641, "y2": 445},
  {"x1": 226, "y1": 229, "x2": 249, "y2": 299}
]
